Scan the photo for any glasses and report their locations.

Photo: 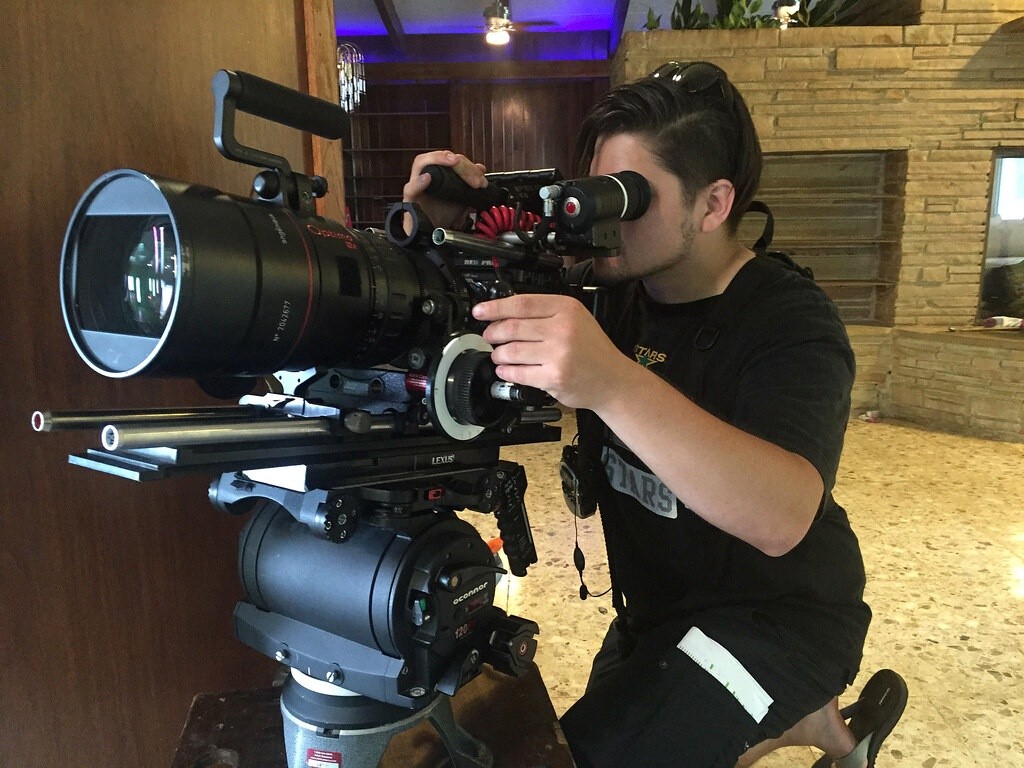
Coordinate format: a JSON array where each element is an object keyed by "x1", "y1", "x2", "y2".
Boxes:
[{"x1": 648, "y1": 61, "x2": 734, "y2": 111}]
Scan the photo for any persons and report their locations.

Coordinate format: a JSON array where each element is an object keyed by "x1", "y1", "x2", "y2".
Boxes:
[{"x1": 401, "y1": 59, "x2": 908, "y2": 768}]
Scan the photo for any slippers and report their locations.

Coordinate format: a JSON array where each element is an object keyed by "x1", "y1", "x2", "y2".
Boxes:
[{"x1": 810, "y1": 669, "x2": 908, "y2": 768}]
